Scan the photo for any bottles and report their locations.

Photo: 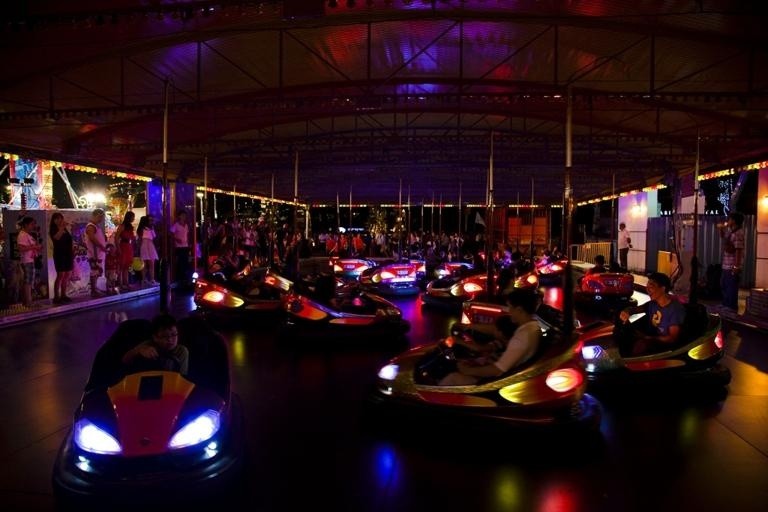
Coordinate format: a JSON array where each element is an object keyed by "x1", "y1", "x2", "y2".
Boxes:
[{"x1": 717, "y1": 221, "x2": 728, "y2": 228}]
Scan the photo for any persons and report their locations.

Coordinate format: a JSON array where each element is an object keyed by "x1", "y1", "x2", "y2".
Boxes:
[
  {"x1": 616, "y1": 271, "x2": 688, "y2": 357},
  {"x1": 454, "y1": 314, "x2": 517, "y2": 369},
  {"x1": 15, "y1": 210, "x2": 191, "y2": 307},
  {"x1": 121, "y1": 312, "x2": 190, "y2": 377},
  {"x1": 310, "y1": 273, "x2": 356, "y2": 311},
  {"x1": 533, "y1": 290, "x2": 559, "y2": 330},
  {"x1": 713, "y1": 211, "x2": 745, "y2": 317},
  {"x1": 617, "y1": 221, "x2": 634, "y2": 272},
  {"x1": 434, "y1": 287, "x2": 542, "y2": 385},
  {"x1": 217, "y1": 251, "x2": 270, "y2": 297},
  {"x1": 195, "y1": 209, "x2": 486, "y2": 263},
  {"x1": 328, "y1": 244, "x2": 635, "y2": 315}
]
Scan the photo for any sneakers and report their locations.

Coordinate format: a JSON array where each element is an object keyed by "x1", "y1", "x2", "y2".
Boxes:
[{"x1": 53, "y1": 296, "x2": 73, "y2": 305}]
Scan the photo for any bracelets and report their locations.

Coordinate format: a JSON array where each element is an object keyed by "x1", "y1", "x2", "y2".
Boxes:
[{"x1": 733, "y1": 265, "x2": 742, "y2": 270}]
